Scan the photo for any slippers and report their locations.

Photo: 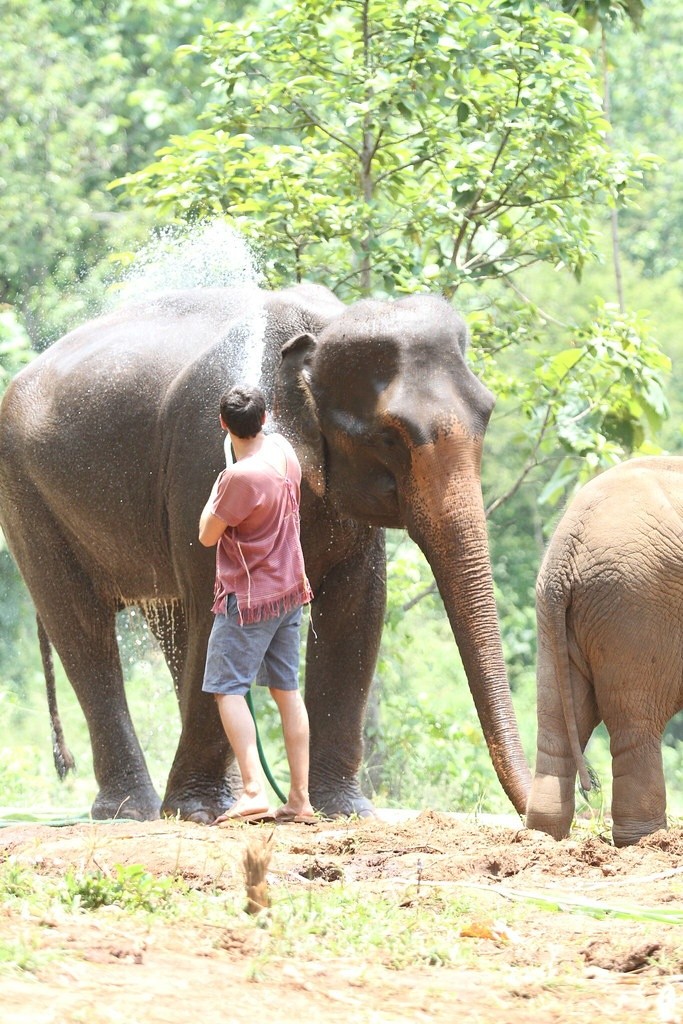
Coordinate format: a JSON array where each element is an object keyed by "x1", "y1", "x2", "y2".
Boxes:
[
  {"x1": 277, "y1": 808, "x2": 317, "y2": 824},
  {"x1": 212, "y1": 804, "x2": 275, "y2": 827}
]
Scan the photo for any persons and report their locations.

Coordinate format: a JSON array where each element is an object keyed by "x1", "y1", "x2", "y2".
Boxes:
[{"x1": 199, "y1": 384, "x2": 318, "y2": 825}]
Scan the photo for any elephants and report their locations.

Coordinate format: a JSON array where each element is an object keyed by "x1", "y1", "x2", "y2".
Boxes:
[{"x1": 0, "y1": 284, "x2": 683, "y2": 851}]
